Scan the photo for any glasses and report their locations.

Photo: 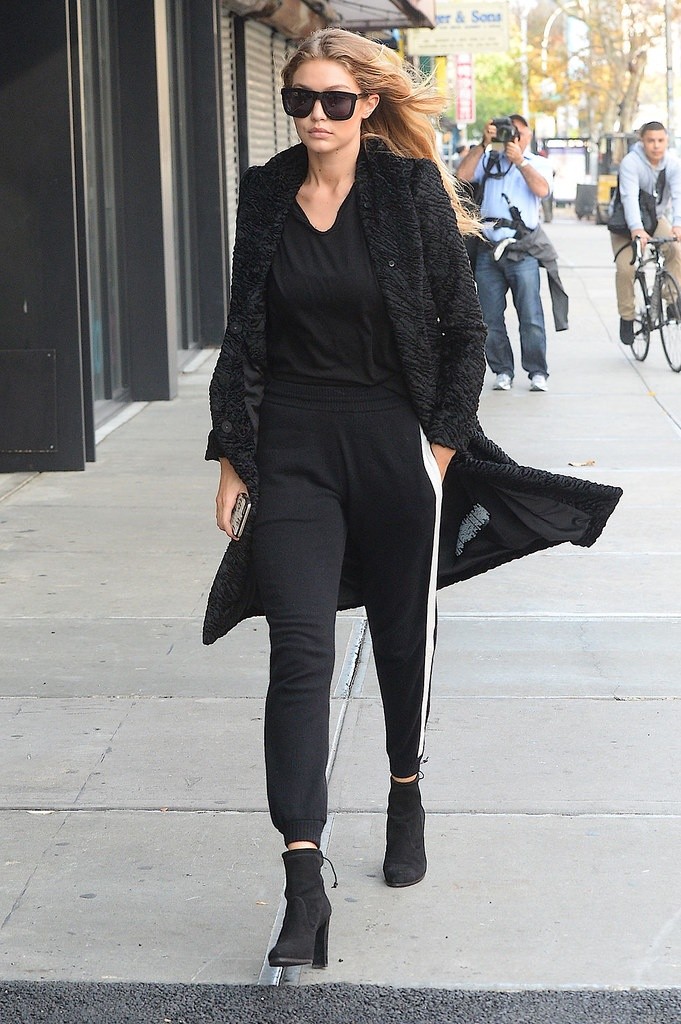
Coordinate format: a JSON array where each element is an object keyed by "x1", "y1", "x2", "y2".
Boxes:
[{"x1": 280, "y1": 88, "x2": 368, "y2": 121}]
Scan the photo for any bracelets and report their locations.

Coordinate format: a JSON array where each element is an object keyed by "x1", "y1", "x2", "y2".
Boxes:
[{"x1": 516, "y1": 158, "x2": 529, "y2": 170}]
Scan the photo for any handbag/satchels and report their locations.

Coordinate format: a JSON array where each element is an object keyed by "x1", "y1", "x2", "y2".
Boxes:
[{"x1": 608, "y1": 186, "x2": 658, "y2": 238}]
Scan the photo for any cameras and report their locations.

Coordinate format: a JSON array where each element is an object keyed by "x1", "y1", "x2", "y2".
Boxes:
[{"x1": 490, "y1": 117, "x2": 517, "y2": 143}]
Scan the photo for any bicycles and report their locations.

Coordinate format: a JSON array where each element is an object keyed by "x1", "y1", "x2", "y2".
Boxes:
[{"x1": 630, "y1": 237, "x2": 681, "y2": 372}]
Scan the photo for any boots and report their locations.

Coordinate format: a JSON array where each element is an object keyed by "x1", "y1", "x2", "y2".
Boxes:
[
  {"x1": 383, "y1": 771, "x2": 427, "y2": 887},
  {"x1": 268, "y1": 847, "x2": 339, "y2": 968}
]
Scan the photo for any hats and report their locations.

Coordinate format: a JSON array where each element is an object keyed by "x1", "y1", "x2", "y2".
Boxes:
[{"x1": 509, "y1": 115, "x2": 528, "y2": 126}]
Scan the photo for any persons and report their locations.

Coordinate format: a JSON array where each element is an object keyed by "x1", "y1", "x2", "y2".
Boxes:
[
  {"x1": 452, "y1": 143, "x2": 487, "y2": 272},
  {"x1": 459, "y1": 114, "x2": 554, "y2": 391},
  {"x1": 206, "y1": 28, "x2": 481, "y2": 972},
  {"x1": 604, "y1": 121, "x2": 681, "y2": 346}
]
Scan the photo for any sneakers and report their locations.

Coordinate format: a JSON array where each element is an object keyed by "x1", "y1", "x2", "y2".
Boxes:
[
  {"x1": 620, "y1": 318, "x2": 634, "y2": 345},
  {"x1": 530, "y1": 375, "x2": 547, "y2": 391},
  {"x1": 492, "y1": 374, "x2": 512, "y2": 390},
  {"x1": 667, "y1": 304, "x2": 681, "y2": 319}
]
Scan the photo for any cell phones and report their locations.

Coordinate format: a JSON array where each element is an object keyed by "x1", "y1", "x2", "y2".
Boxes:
[{"x1": 230, "y1": 493, "x2": 251, "y2": 538}]
